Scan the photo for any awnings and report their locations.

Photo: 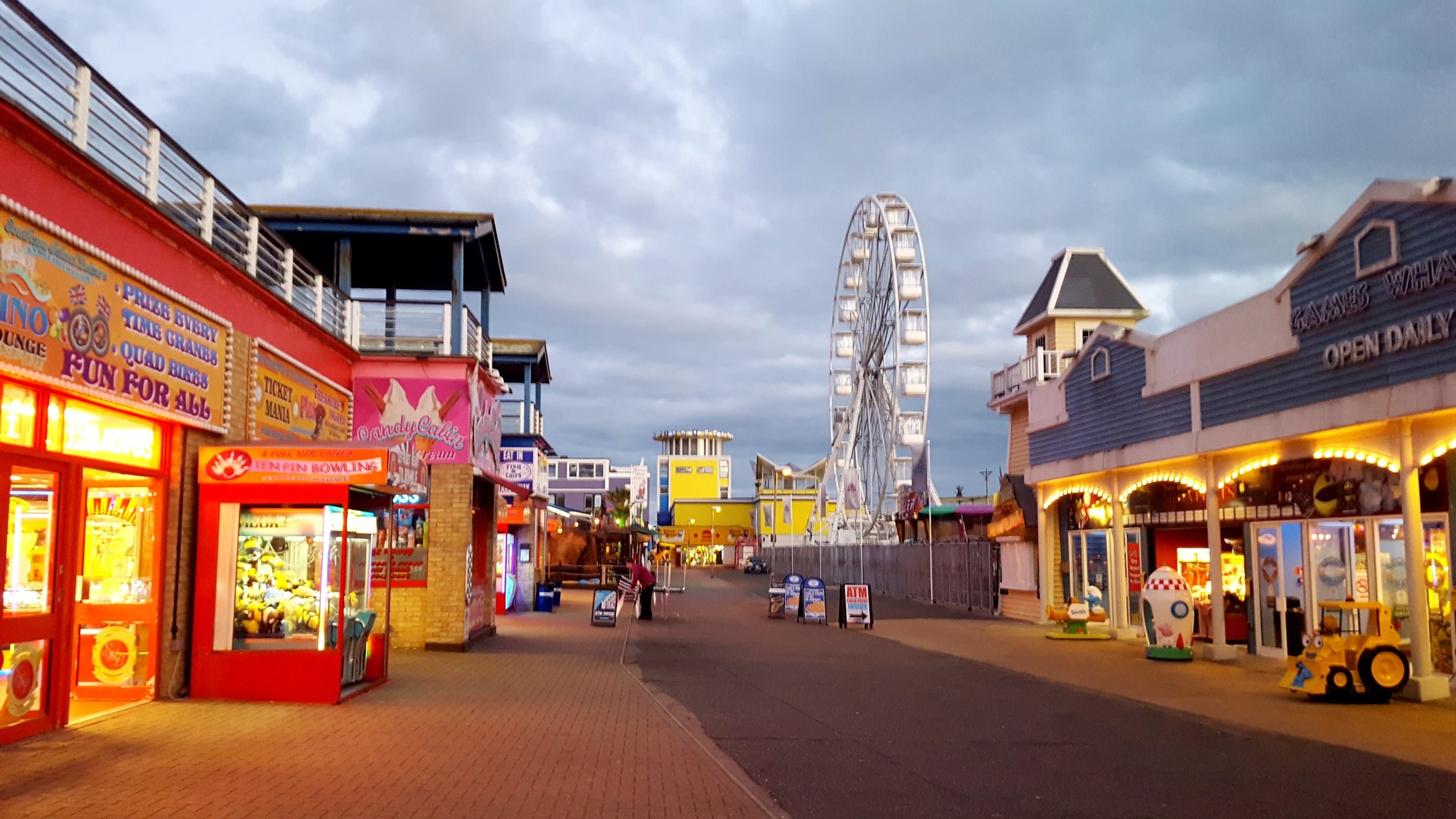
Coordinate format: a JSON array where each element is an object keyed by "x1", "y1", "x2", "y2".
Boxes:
[{"x1": 658, "y1": 525, "x2": 745, "y2": 545}]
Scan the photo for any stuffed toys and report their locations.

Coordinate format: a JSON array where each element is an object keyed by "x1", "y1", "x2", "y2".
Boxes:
[{"x1": 234, "y1": 539, "x2": 358, "y2": 634}]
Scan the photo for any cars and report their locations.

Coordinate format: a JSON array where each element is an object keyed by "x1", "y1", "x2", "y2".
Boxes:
[
  {"x1": 1280, "y1": 594, "x2": 1410, "y2": 704},
  {"x1": 743, "y1": 555, "x2": 768, "y2": 575}
]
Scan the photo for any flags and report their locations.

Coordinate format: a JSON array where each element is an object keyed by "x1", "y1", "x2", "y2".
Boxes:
[
  {"x1": 843, "y1": 468, "x2": 860, "y2": 509},
  {"x1": 782, "y1": 496, "x2": 792, "y2": 523},
  {"x1": 821, "y1": 483, "x2": 826, "y2": 517},
  {"x1": 749, "y1": 509, "x2": 756, "y2": 528},
  {"x1": 911, "y1": 443, "x2": 928, "y2": 493},
  {"x1": 764, "y1": 503, "x2": 772, "y2": 526}
]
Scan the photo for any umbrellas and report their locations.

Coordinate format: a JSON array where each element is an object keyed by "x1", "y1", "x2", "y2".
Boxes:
[{"x1": 630, "y1": 523, "x2": 659, "y2": 554}]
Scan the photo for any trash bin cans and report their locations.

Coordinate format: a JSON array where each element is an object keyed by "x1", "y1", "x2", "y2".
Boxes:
[
  {"x1": 546, "y1": 579, "x2": 562, "y2": 605},
  {"x1": 535, "y1": 583, "x2": 554, "y2": 611}
]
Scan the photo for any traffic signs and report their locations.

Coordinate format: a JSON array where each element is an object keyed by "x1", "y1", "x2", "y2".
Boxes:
[{"x1": 845, "y1": 584, "x2": 870, "y2": 624}]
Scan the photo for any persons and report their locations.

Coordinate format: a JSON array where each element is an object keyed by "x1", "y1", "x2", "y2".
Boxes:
[
  {"x1": 625, "y1": 557, "x2": 656, "y2": 620},
  {"x1": 1084, "y1": 586, "x2": 1102, "y2": 610}
]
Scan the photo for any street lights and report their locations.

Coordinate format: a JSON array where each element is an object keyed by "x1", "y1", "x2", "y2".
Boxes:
[
  {"x1": 770, "y1": 465, "x2": 793, "y2": 584},
  {"x1": 688, "y1": 518, "x2": 695, "y2": 569},
  {"x1": 629, "y1": 499, "x2": 645, "y2": 546},
  {"x1": 711, "y1": 506, "x2": 722, "y2": 578}
]
